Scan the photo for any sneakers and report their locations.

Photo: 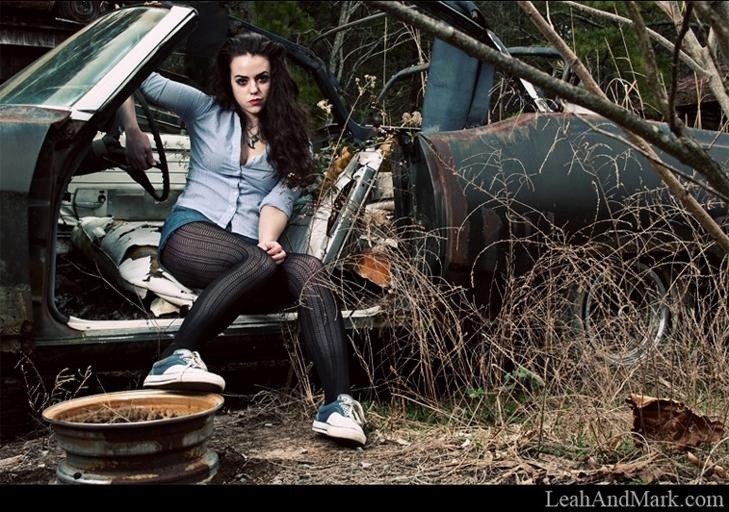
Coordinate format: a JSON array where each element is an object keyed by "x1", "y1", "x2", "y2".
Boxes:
[
  {"x1": 143, "y1": 348, "x2": 225, "y2": 391},
  {"x1": 312, "y1": 394, "x2": 366, "y2": 447}
]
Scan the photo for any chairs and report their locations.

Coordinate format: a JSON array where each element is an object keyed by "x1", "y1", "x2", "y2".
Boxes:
[{"x1": 80, "y1": 146, "x2": 383, "y2": 313}]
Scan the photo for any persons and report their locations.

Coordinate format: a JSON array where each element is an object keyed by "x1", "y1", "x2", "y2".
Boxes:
[
  {"x1": 119, "y1": 97, "x2": 152, "y2": 172},
  {"x1": 140, "y1": 33, "x2": 367, "y2": 445}
]
